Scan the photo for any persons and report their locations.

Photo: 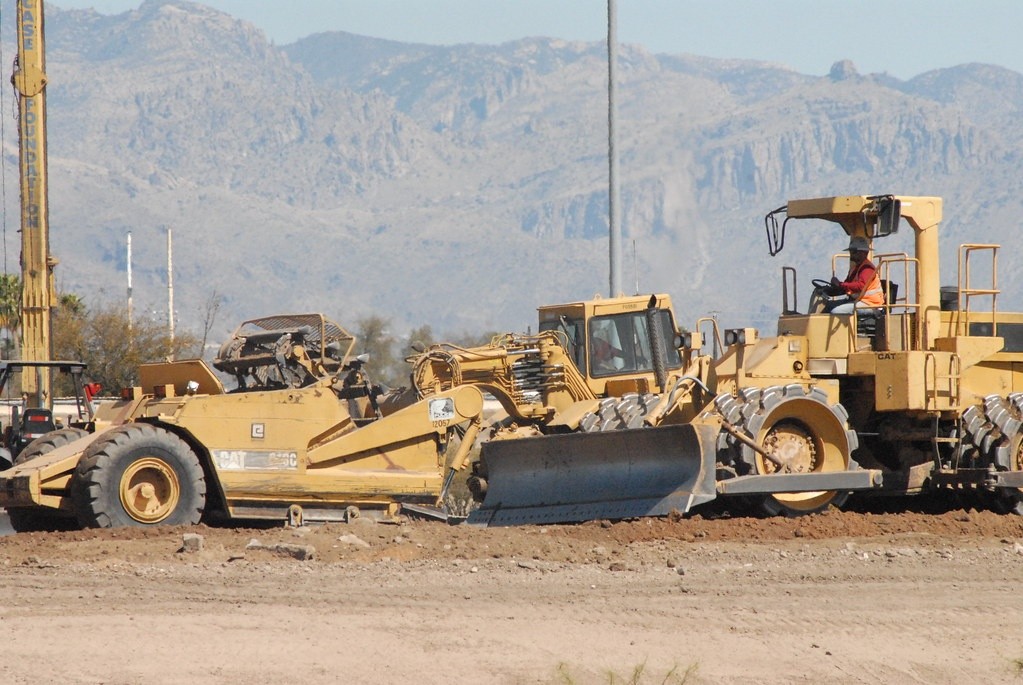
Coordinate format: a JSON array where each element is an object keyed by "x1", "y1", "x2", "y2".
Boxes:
[
  {"x1": 567, "y1": 319, "x2": 648, "y2": 373},
  {"x1": 807, "y1": 235, "x2": 886, "y2": 317}
]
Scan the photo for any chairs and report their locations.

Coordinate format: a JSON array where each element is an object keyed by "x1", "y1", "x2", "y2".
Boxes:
[{"x1": 860, "y1": 281, "x2": 898, "y2": 329}]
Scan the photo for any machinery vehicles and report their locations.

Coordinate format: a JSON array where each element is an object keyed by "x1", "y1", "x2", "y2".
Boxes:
[
  {"x1": 466, "y1": 195, "x2": 1023, "y2": 525},
  {"x1": 0, "y1": 1, "x2": 95, "y2": 471},
  {"x1": 0, "y1": 293, "x2": 724, "y2": 531}
]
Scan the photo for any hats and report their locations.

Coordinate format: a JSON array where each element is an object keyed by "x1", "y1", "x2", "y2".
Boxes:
[{"x1": 842, "y1": 237, "x2": 874, "y2": 250}]
